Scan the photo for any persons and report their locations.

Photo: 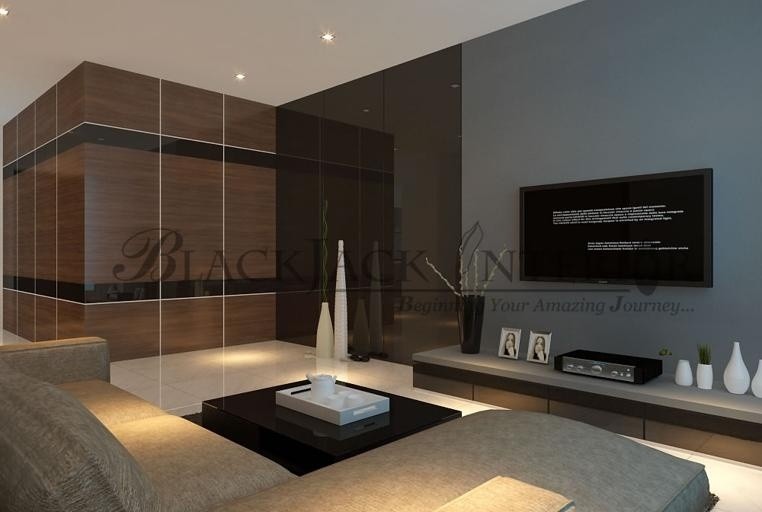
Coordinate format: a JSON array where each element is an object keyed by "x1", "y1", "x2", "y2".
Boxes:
[
  {"x1": 502, "y1": 331, "x2": 516, "y2": 356},
  {"x1": 530, "y1": 335, "x2": 546, "y2": 361}
]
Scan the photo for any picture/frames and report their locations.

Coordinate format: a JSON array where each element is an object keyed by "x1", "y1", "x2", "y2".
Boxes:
[
  {"x1": 498, "y1": 326, "x2": 522, "y2": 360},
  {"x1": 526, "y1": 328, "x2": 552, "y2": 365}
]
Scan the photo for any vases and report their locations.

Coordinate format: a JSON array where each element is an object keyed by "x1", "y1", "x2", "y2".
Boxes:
[
  {"x1": 455, "y1": 295, "x2": 484, "y2": 355},
  {"x1": 314, "y1": 240, "x2": 348, "y2": 360},
  {"x1": 723, "y1": 341, "x2": 762, "y2": 399}
]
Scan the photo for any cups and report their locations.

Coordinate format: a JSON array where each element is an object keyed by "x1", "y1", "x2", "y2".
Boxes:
[{"x1": 327, "y1": 389, "x2": 364, "y2": 410}]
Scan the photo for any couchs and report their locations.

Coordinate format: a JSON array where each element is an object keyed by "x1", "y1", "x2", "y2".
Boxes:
[{"x1": 0, "y1": 336, "x2": 720, "y2": 511}]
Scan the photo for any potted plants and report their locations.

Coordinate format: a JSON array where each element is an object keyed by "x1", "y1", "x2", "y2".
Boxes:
[{"x1": 696, "y1": 343, "x2": 714, "y2": 389}]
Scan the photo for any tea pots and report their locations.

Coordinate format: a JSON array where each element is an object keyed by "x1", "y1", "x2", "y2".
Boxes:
[{"x1": 306, "y1": 372, "x2": 337, "y2": 402}]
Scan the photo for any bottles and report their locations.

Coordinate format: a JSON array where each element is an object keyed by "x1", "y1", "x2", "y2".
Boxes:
[
  {"x1": 751, "y1": 359, "x2": 762, "y2": 398},
  {"x1": 722, "y1": 340, "x2": 750, "y2": 395}
]
